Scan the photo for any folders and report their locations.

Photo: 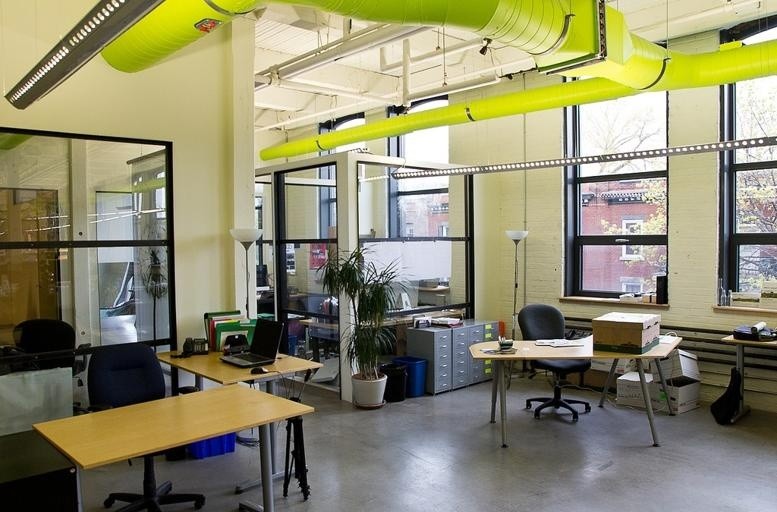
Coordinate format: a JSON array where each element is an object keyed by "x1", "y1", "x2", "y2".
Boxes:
[{"x1": 204, "y1": 310, "x2": 256, "y2": 352}]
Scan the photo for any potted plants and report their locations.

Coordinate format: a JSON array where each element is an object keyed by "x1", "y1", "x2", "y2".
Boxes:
[{"x1": 315, "y1": 238, "x2": 414, "y2": 409}]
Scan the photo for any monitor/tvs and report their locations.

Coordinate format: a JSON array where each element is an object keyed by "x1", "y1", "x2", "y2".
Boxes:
[{"x1": 381, "y1": 280, "x2": 420, "y2": 311}]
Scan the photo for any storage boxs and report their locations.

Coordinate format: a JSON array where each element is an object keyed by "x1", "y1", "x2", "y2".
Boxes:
[
  {"x1": 592, "y1": 311, "x2": 662, "y2": 355},
  {"x1": 616, "y1": 371, "x2": 653, "y2": 408},
  {"x1": 648, "y1": 349, "x2": 703, "y2": 383},
  {"x1": 649, "y1": 380, "x2": 701, "y2": 415}
]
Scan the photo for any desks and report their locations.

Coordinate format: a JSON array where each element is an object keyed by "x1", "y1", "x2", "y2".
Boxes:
[
  {"x1": 155, "y1": 347, "x2": 325, "y2": 495},
  {"x1": 31, "y1": 383, "x2": 316, "y2": 512},
  {"x1": 468, "y1": 335, "x2": 682, "y2": 448},
  {"x1": 298, "y1": 311, "x2": 466, "y2": 374},
  {"x1": 720, "y1": 334, "x2": 777, "y2": 424}
]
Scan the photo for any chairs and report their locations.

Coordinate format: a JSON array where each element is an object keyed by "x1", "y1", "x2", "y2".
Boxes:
[
  {"x1": 12, "y1": 317, "x2": 91, "y2": 414},
  {"x1": 517, "y1": 304, "x2": 593, "y2": 422},
  {"x1": 87, "y1": 343, "x2": 207, "y2": 512}
]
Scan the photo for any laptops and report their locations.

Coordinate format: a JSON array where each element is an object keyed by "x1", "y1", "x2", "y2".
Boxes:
[{"x1": 219, "y1": 317, "x2": 284, "y2": 369}]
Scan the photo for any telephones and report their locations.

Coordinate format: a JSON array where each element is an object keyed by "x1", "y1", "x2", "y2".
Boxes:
[{"x1": 169, "y1": 337, "x2": 209, "y2": 359}]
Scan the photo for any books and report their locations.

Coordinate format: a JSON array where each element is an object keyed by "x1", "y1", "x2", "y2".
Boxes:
[
  {"x1": 204, "y1": 314, "x2": 250, "y2": 352},
  {"x1": 431, "y1": 317, "x2": 460, "y2": 325}
]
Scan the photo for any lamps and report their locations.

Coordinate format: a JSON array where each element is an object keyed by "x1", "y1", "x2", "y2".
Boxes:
[
  {"x1": 480, "y1": 46, "x2": 488, "y2": 55},
  {"x1": 505, "y1": 230, "x2": 528, "y2": 339},
  {"x1": 229, "y1": 227, "x2": 265, "y2": 320}
]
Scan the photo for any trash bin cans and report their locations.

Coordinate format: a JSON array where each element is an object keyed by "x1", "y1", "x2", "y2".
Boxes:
[
  {"x1": 393, "y1": 356, "x2": 428, "y2": 398},
  {"x1": 380, "y1": 363, "x2": 408, "y2": 403}
]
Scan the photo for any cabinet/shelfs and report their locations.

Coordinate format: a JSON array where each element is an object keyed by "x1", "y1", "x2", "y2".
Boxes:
[{"x1": 406, "y1": 319, "x2": 500, "y2": 396}]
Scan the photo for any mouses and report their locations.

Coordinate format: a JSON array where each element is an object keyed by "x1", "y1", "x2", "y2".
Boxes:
[{"x1": 250, "y1": 366, "x2": 270, "y2": 374}]
